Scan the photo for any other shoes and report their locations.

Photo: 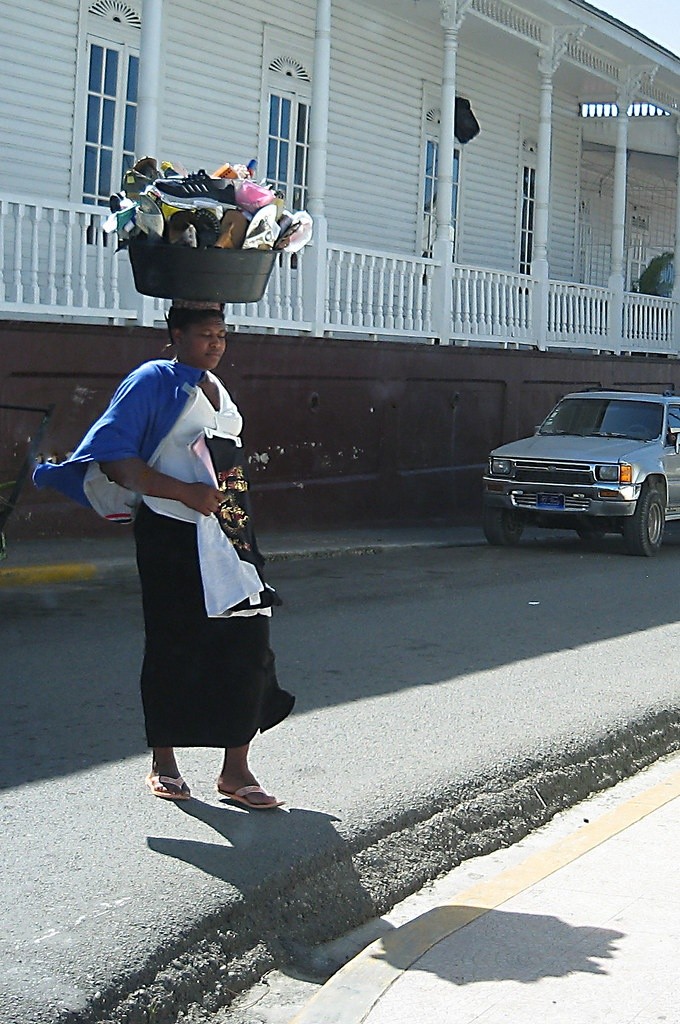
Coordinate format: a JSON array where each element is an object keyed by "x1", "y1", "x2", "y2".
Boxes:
[{"x1": 101, "y1": 155, "x2": 313, "y2": 254}]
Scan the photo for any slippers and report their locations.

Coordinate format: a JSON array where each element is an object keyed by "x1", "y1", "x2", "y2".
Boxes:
[
  {"x1": 146, "y1": 771, "x2": 190, "y2": 799},
  {"x1": 218, "y1": 785, "x2": 286, "y2": 809}
]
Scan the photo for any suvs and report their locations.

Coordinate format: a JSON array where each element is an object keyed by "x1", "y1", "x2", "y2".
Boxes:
[{"x1": 481, "y1": 387, "x2": 680, "y2": 557}]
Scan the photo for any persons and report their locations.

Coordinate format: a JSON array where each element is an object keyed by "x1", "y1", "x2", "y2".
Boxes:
[{"x1": 30, "y1": 308, "x2": 295, "y2": 809}]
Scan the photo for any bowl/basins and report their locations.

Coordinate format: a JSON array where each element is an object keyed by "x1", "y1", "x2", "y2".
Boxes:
[{"x1": 129, "y1": 238, "x2": 284, "y2": 303}]
